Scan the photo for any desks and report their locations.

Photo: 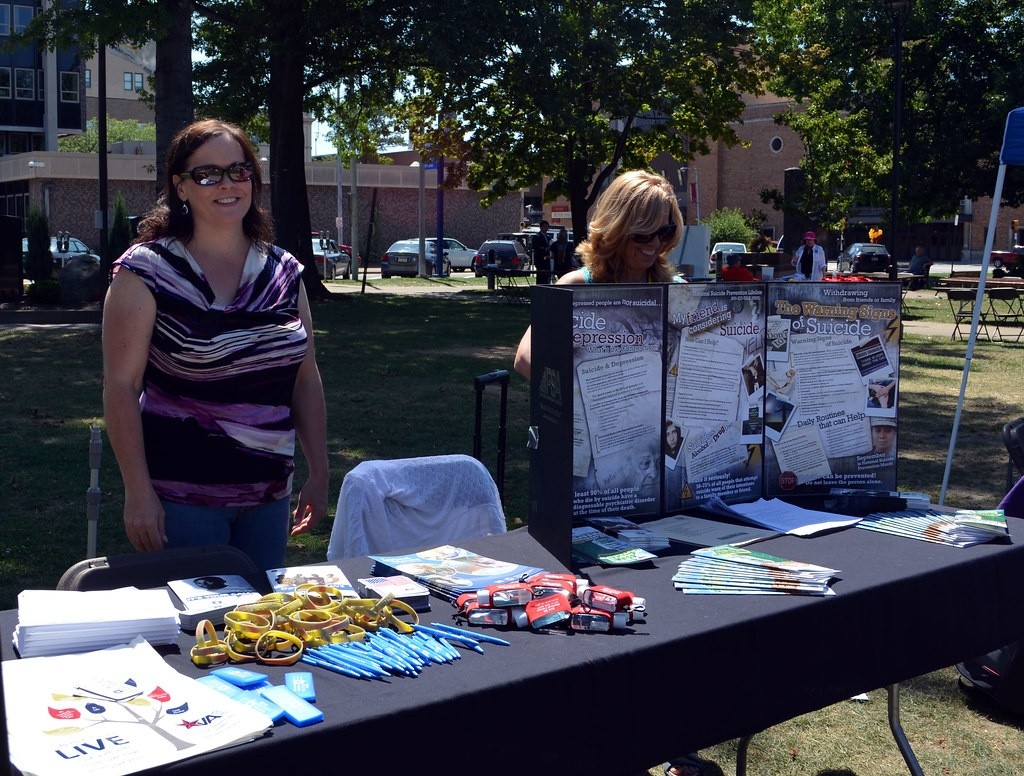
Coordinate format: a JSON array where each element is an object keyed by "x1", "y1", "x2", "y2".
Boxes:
[
  {"x1": 867, "y1": 275, "x2": 925, "y2": 314},
  {"x1": 935, "y1": 277, "x2": 1024, "y2": 296},
  {"x1": 0, "y1": 489, "x2": 1024, "y2": 776}
]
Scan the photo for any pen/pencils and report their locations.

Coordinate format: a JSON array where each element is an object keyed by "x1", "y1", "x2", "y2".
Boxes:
[
  {"x1": 456, "y1": 633, "x2": 484, "y2": 654},
  {"x1": 299, "y1": 626, "x2": 461, "y2": 679},
  {"x1": 410, "y1": 624, "x2": 479, "y2": 646},
  {"x1": 430, "y1": 622, "x2": 511, "y2": 647}
]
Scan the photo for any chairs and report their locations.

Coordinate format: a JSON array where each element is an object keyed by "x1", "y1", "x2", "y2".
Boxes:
[
  {"x1": 495, "y1": 269, "x2": 557, "y2": 289},
  {"x1": 898, "y1": 272, "x2": 914, "y2": 314},
  {"x1": 325, "y1": 455, "x2": 506, "y2": 561},
  {"x1": 948, "y1": 290, "x2": 992, "y2": 342},
  {"x1": 987, "y1": 287, "x2": 1024, "y2": 342}
]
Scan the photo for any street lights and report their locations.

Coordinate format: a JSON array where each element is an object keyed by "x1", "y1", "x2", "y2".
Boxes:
[{"x1": 882, "y1": 0, "x2": 916, "y2": 279}]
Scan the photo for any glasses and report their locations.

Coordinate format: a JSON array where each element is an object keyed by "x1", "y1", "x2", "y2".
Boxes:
[
  {"x1": 180, "y1": 162, "x2": 254, "y2": 185},
  {"x1": 628, "y1": 222, "x2": 677, "y2": 244}
]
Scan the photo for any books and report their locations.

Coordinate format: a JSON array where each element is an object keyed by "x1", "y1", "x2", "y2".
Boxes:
[
  {"x1": 12, "y1": 588, "x2": 183, "y2": 656},
  {"x1": 265, "y1": 564, "x2": 364, "y2": 604},
  {"x1": 167, "y1": 571, "x2": 264, "y2": 630}
]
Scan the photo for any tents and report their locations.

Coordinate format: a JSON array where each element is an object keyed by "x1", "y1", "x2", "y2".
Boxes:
[{"x1": 937, "y1": 104, "x2": 1023, "y2": 510}]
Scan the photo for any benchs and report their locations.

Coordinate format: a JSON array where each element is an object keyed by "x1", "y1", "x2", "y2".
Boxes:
[{"x1": 932, "y1": 287, "x2": 990, "y2": 296}]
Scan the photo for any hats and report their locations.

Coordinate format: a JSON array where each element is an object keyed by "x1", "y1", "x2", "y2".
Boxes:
[
  {"x1": 804, "y1": 231, "x2": 816, "y2": 240},
  {"x1": 726, "y1": 253, "x2": 741, "y2": 265}
]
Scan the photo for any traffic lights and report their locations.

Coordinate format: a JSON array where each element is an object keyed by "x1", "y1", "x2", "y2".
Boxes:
[{"x1": 1011, "y1": 220, "x2": 1018, "y2": 231}]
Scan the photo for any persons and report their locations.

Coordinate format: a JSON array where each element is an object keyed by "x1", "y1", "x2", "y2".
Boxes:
[
  {"x1": 531, "y1": 221, "x2": 551, "y2": 285},
  {"x1": 792, "y1": 231, "x2": 828, "y2": 282},
  {"x1": 511, "y1": 169, "x2": 726, "y2": 776},
  {"x1": 663, "y1": 418, "x2": 683, "y2": 461},
  {"x1": 869, "y1": 416, "x2": 897, "y2": 458},
  {"x1": 543, "y1": 227, "x2": 574, "y2": 279},
  {"x1": 908, "y1": 244, "x2": 934, "y2": 284},
  {"x1": 623, "y1": 433, "x2": 659, "y2": 503},
  {"x1": 721, "y1": 252, "x2": 764, "y2": 282},
  {"x1": 99, "y1": 118, "x2": 331, "y2": 587},
  {"x1": 868, "y1": 377, "x2": 894, "y2": 410}
]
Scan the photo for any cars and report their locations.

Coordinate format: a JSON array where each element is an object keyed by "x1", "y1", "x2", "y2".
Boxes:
[
  {"x1": 311, "y1": 238, "x2": 364, "y2": 281},
  {"x1": 709, "y1": 242, "x2": 747, "y2": 274},
  {"x1": 23, "y1": 235, "x2": 101, "y2": 295},
  {"x1": 770, "y1": 240, "x2": 783, "y2": 252},
  {"x1": 380, "y1": 240, "x2": 452, "y2": 278},
  {"x1": 496, "y1": 223, "x2": 580, "y2": 274},
  {"x1": 836, "y1": 243, "x2": 892, "y2": 274},
  {"x1": 989, "y1": 249, "x2": 1021, "y2": 268}
]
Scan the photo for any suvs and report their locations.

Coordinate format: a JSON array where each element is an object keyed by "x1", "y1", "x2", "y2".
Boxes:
[
  {"x1": 412, "y1": 238, "x2": 479, "y2": 273},
  {"x1": 474, "y1": 239, "x2": 531, "y2": 278}
]
